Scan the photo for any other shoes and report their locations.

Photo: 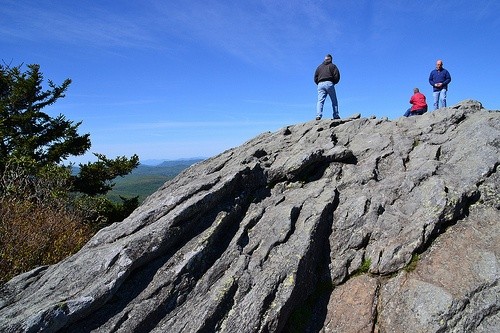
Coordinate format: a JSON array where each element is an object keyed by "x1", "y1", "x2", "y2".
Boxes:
[{"x1": 316, "y1": 117, "x2": 321, "y2": 121}]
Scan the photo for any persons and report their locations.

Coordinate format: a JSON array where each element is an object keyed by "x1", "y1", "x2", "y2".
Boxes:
[
  {"x1": 403, "y1": 88, "x2": 428, "y2": 118},
  {"x1": 429, "y1": 59, "x2": 452, "y2": 110},
  {"x1": 313, "y1": 53, "x2": 341, "y2": 120}
]
